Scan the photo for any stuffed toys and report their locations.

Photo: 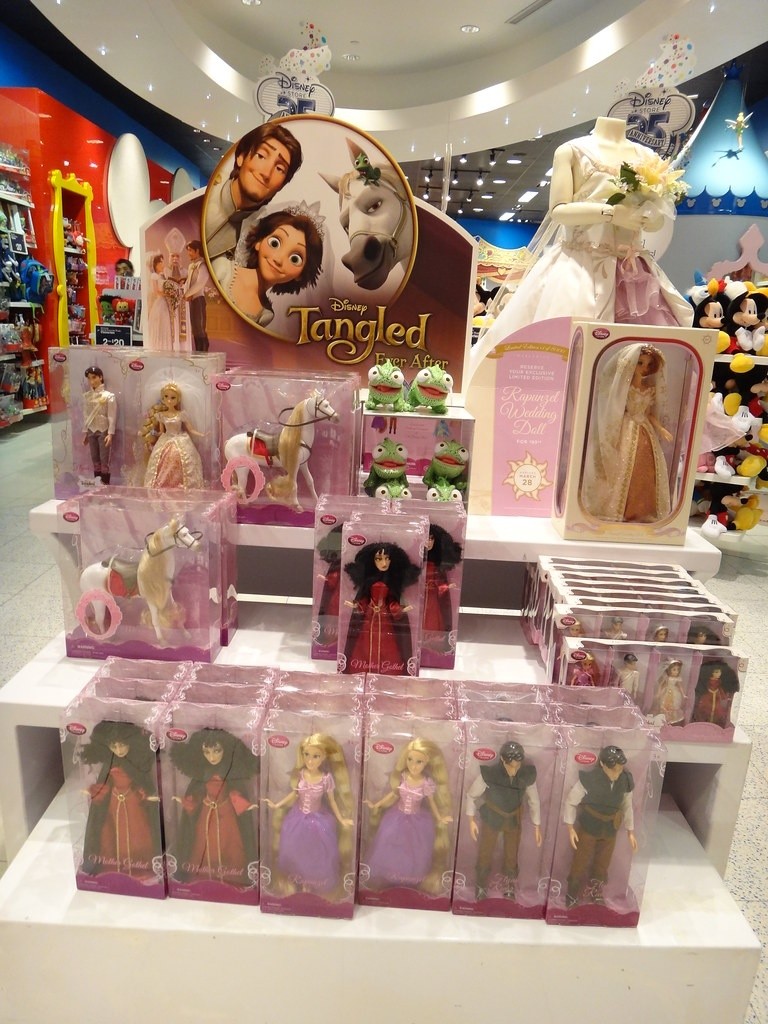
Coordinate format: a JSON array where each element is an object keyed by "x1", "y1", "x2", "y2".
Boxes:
[
  {"x1": 690, "y1": 279, "x2": 768, "y2": 534},
  {"x1": 365, "y1": 360, "x2": 469, "y2": 503},
  {"x1": 473, "y1": 284, "x2": 516, "y2": 318}
]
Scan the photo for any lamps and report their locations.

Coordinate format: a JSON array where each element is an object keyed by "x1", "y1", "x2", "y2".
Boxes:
[{"x1": 418, "y1": 149, "x2": 505, "y2": 215}]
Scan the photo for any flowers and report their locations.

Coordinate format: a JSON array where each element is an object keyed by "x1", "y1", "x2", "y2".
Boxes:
[{"x1": 605, "y1": 152, "x2": 692, "y2": 208}]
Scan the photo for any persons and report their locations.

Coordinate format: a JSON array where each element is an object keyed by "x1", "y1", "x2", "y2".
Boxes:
[
  {"x1": 563, "y1": 745, "x2": 638, "y2": 906},
  {"x1": 25, "y1": 366, "x2": 45, "y2": 399},
  {"x1": 315, "y1": 525, "x2": 341, "y2": 644},
  {"x1": 116, "y1": 259, "x2": 134, "y2": 276},
  {"x1": 130, "y1": 384, "x2": 211, "y2": 490},
  {"x1": 558, "y1": 615, "x2": 740, "y2": 728},
  {"x1": 81, "y1": 367, "x2": 117, "y2": 484},
  {"x1": 467, "y1": 742, "x2": 542, "y2": 900},
  {"x1": 79, "y1": 721, "x2": 161, "y2": 878},
  {"x1": 99, "y1": 295, "x2": 135, "y2": 325},
  {"x1": 589, "y1": 343, "x2": 674, "y2": 521},
  {"x1": 469, "y1": 117, "x2": 695, "y2": 375},
  {"x1": 345, "y1": 543, "x2": 417, "y2": 674},
  {"x1": 363, "y1": 737, "x2": 453, "y2": 894},
  {"x1": 265, "y1": 733, "x2": 355, "y2": 899},
  {"x1": 421, "y1": 525, "x2": 463, "y2": 629},
  {"x1": 170, "y1": 728, "x2": 260, "y2": 883}
]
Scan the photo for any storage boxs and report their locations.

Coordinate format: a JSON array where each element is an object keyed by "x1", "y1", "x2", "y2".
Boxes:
[{"x1": 46, "y1": 320, "x2": 750, "y2": 927}]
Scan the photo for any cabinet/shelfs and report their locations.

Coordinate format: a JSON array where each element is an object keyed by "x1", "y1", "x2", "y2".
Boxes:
[
  {"x1": 0, "y1": 499, "x2": 768, "y2": 1024},
  {"x1": 89, "y1": 288, "x2": 143, "y2": 342},
  {"x1": 695, "y1": 354, "x2": 768, "y2": 551},
  {"x1": 64, "y1": 246, "x2": 88, "y2": 345},
  {"x1": 0, "y1": 162, "x2": 47, "y2": 428}
]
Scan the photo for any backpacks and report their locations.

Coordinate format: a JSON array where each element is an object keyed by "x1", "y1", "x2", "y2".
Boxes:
[{"x1": 19, "y1": 254, "x2": 55, "y2": 319}]
